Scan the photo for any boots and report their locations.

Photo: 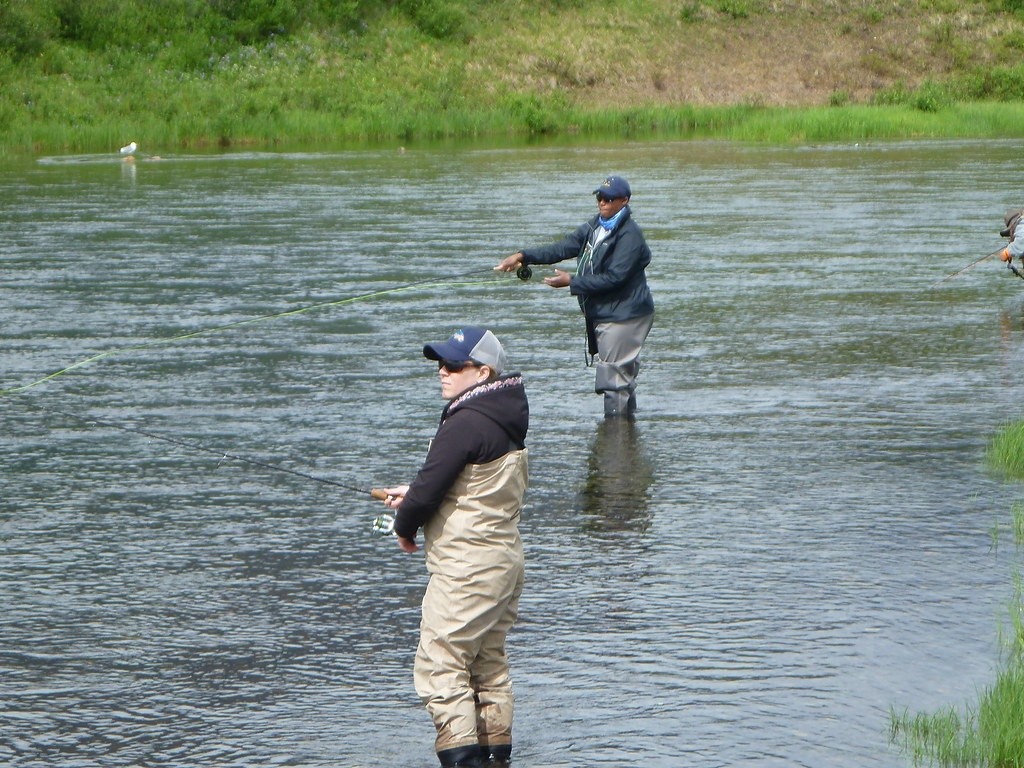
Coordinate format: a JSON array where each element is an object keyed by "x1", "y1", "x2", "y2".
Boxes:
[{"x1": 436, "y1": 739, "x2": 512, "y2": 768}]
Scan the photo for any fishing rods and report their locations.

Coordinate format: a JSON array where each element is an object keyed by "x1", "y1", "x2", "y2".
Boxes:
[
  {"x1": 66, "y1": 408, "x2": 406, "y2": 544},
  {"x1": 161, "y1": 262, "x2": 537, "y2": 344}
]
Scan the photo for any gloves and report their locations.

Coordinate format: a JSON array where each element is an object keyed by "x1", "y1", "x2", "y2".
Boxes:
[{"x1": 1000, "y1": 249, "x2": 1011, "y2": 262}]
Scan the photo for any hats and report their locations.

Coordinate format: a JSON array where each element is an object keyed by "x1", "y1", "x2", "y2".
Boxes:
[
  {"x1": 423, "y1": 327, "x2": 506, "y2": 374},
  {"x1": 1000, "y1": 208, "x2": 1024, "y2": 237},
  {"x1": 592, "y1": 176, "x2": 631, "y2": 196}
]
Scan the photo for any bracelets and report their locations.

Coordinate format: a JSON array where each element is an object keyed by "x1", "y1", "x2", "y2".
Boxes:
[{"x1": 1004, "y1": 250, "x2": 1008, "y2": 257}]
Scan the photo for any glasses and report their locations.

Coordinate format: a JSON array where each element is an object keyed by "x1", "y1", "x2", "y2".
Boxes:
[
  {"x1": 596, "y1": 194, "x2": 626, "y2": 203},
  {"x1": 439, "y1": 358, "x2": 485, "y2": 372}
]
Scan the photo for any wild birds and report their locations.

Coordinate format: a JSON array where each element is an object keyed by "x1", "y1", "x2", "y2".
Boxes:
[{"x1": 120, "y1": 141, "x2": 137, "y2": 153}]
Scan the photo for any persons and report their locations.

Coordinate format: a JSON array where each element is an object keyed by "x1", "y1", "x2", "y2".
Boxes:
[
  {"x1": 999, "y1": 208, "x2": 1024, "y2": 268},
  {"x1": 499, "y1": 176, "x2": 655, "y2": 415},
  {"x1": 385, "y1": 326, "x2": 529, "y2": 768}
]
